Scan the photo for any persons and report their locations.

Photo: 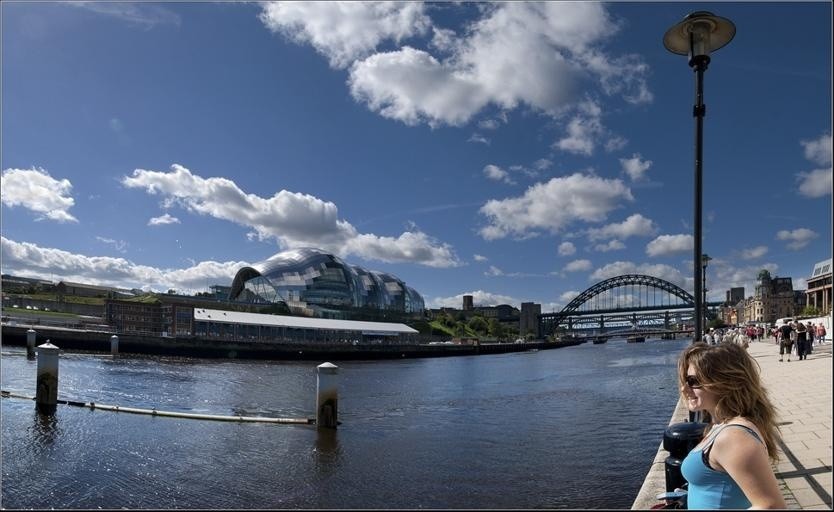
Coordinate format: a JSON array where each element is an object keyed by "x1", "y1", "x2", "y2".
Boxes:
[
  {"x1": 701, "y1": 320, "x2": 826, "y2": 362},
  {"x1": 664, "y1": 341, "x2": 788, "y2": 510}
]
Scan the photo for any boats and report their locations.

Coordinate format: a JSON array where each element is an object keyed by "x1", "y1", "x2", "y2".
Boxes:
[
  {"x1": 628, "y1": 336, "x2": 644, "y2": 343},
  {"x1": 593, "y1": 338, "x2": 608, "y2": 344}
]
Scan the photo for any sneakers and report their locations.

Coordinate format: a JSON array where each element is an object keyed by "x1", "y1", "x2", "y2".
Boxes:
[
  {"x1": 799, "y1": 355, "x2": 806, "y2": 360},
  {"x1": 779, "y1": 359, "x2": 791, "y2": 362}
]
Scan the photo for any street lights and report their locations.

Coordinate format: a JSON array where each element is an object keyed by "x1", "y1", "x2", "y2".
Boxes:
[{"x1": 662, "y1": 10, "x2": 736, "y2": 343}]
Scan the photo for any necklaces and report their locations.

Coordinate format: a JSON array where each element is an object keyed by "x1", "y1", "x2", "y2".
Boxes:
[{"x1": 702, "y1": 418, "x2": 725, "y2": 442}]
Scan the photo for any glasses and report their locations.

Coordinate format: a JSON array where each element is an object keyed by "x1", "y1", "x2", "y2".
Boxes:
[{"x1": 685, "y1": 375, "x2": 703, "y2": 389}]
